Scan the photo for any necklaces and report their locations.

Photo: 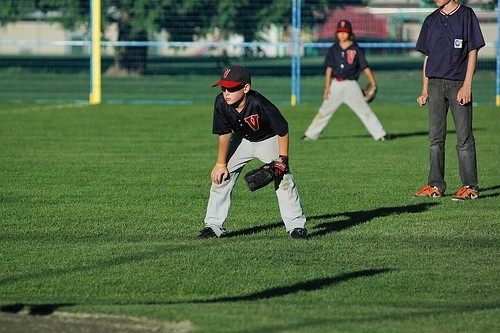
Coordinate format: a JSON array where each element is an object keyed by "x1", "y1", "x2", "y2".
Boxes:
[{"x1": 440, "y1": 3, "x2": 460, "y2": 15}]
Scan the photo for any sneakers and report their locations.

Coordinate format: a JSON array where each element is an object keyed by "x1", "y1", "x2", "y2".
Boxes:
[
  {"x1": 291, "y1": 228, "x2": 307, "y2": 239},
  {"x1": 451, "y1": 186, "x2": 478, "y2": 201},
  {"x1": 197, "y1": 227, "x2": 225, "y2": 239},
  {"x1": 415, "y1": 185, "x2": 444, "y2": 197}
]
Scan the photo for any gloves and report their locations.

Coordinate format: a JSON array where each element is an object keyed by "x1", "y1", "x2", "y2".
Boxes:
[{"x1": 274, "y1": 156, "x2": 288, "y2": 175}]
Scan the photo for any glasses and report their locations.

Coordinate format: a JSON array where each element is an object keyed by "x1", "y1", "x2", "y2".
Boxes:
[{"x1": 221, "y1": 84, "x2": 245, "y2": 92}]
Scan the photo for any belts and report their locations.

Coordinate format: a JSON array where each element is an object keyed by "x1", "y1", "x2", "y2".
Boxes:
[{"x1": 335, "y1": 77, "x2": 345, "y2": 81}]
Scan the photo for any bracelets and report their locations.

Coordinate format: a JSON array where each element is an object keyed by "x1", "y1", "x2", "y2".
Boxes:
[{"x1": 216, "y1": 163, "x2": 226, "y2": 167}]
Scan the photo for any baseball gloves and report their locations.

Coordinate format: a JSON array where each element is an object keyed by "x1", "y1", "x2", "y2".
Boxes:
[
  {"x1": 361, "y1": 82, "x2": 378, "y2": 102},
  {"x1": 243, "y1": 163, "x2": 281, "y2": 193}
]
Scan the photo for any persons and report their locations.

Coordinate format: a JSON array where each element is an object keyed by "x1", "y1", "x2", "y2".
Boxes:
[
  {"x1": 303, "y1": 20, "x2": 387, "y2": 140},
  {"x1": 198, "y1": 66, "x2": 309, "y2": 239},
  {"x1": 416, "y1": 0, "x2": 486, "y2": 199}
]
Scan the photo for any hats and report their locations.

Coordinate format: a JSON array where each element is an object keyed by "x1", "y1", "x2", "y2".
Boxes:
[
  {"x1": 336, "y1": 20, "x2": 351, "y2": 33},
  {"x1": 212, "y1": 64, "x2": 250, "y2": 88}
]
